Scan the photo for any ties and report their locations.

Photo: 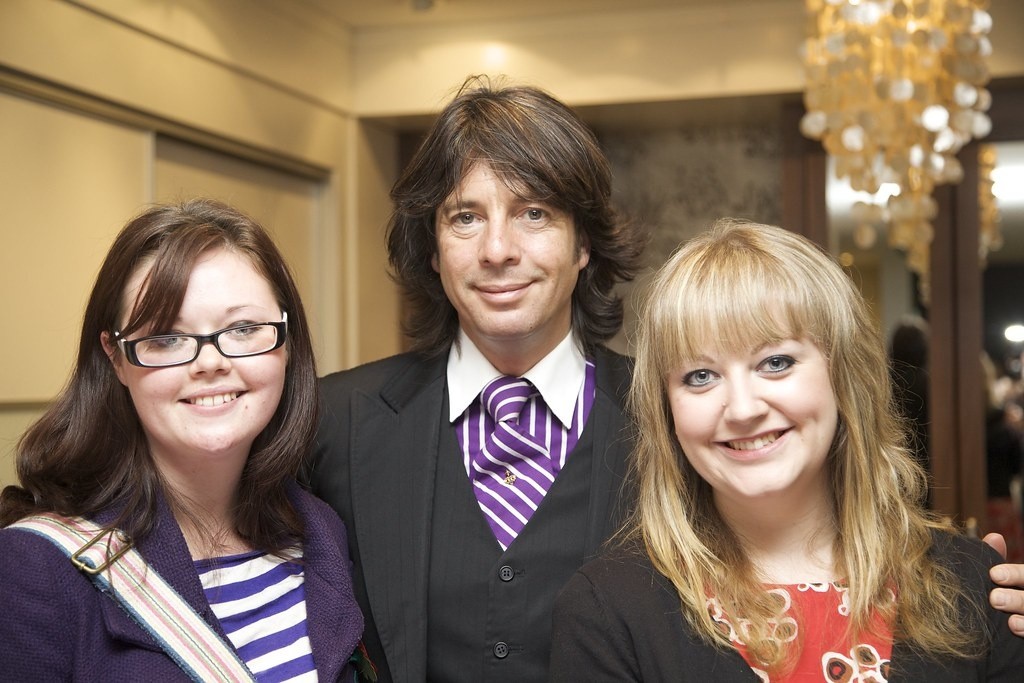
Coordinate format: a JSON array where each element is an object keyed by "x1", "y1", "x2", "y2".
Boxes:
[{"x1": 468, "y1": 375, "x2": 556, "y2": 552}]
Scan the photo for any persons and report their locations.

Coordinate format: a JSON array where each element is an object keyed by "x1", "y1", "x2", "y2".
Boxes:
[
  {"x1": 290, "y1": 86, "x2": 1024, "y2": 683},
  {"x1": 2, "y1": 200, "x2": 373, "y2": 683},
  {"x1": 546, "y1": 216, "x2": 1024, "y2": 683}
]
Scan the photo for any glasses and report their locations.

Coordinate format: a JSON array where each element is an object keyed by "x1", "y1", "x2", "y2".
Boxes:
[{"x1": 116, "y1": 311, "x2": 288, "y2": 368}]
